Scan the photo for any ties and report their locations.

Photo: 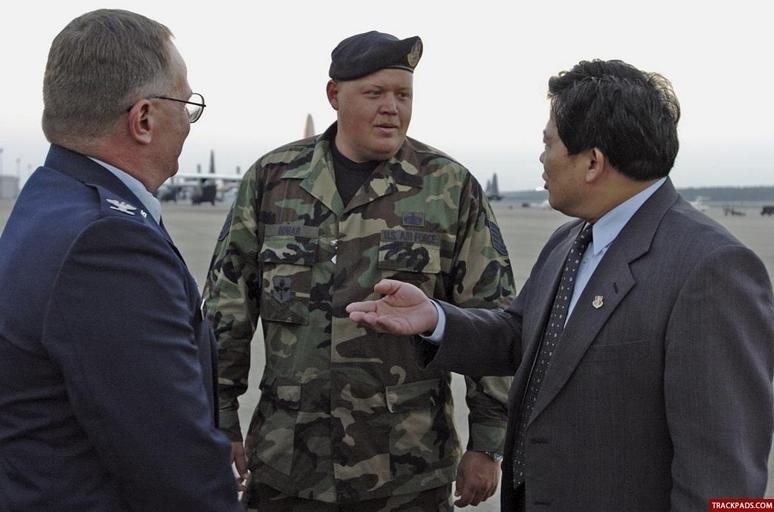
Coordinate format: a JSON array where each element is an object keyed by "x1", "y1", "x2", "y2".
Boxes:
[{"x1": 511, "y1": 225, "x2": 597, "y2": 488}]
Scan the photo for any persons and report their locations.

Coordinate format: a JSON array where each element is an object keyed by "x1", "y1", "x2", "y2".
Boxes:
[
  {"x1": 0, "y1": 9, "x2": 244, "y2": 512},
  {"x1": 346, "y1": 57, "x2": 772, "y2": 511},
  {"x1": 196, "y1": 29, "x2": 518, "y2": 511}
]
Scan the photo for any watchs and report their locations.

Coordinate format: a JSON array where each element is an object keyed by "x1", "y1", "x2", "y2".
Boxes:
[{"x1": 482, "y1": 452, "x2": 504, "y2": 463}]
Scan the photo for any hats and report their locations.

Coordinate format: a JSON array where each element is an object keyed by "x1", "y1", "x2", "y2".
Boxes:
[{"x1": 327, "y1": 31, "x2": 425, "y2": 81}]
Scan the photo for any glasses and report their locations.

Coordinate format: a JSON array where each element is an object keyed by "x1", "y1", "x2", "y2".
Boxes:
[{"x1": 128, "y1": 90, "x2": 207, "y2": 123}]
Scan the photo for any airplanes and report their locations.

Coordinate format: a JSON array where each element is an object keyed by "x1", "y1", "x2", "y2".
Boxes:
[{"x1": 160, "y1": 150, "x2": 242, "y2": 206}]
